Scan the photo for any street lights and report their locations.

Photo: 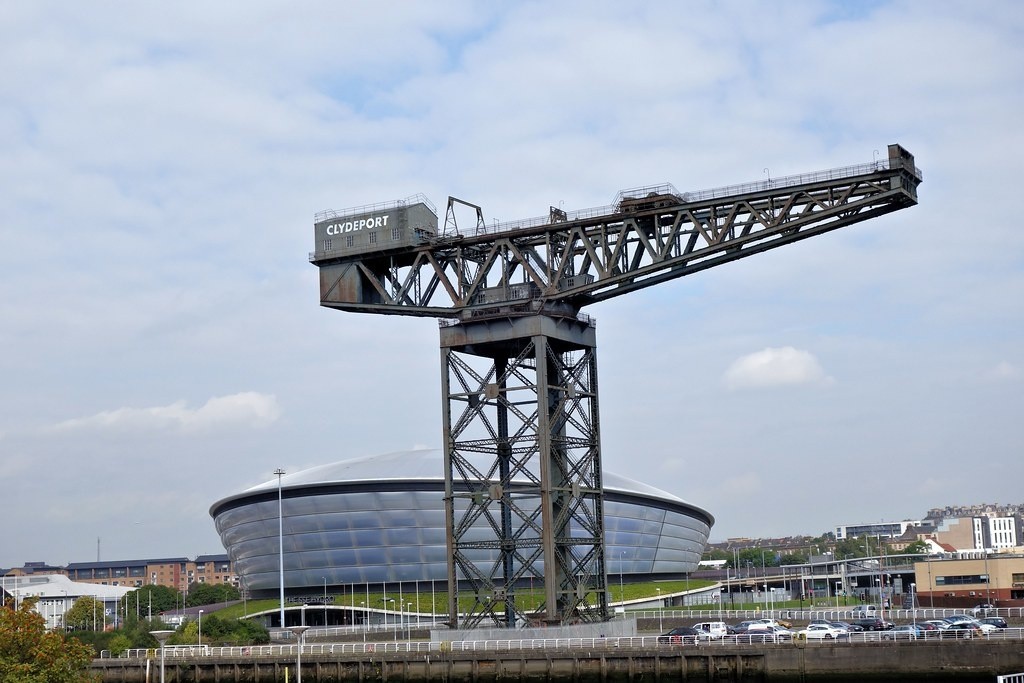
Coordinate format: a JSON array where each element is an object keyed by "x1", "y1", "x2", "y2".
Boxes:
[
  {"x1": 389, "y1": 599, "x2": 396, "y2": 643},
  {"x1": 810, "y1": 544, "x2": 816, "y2": 575},
  {"x1": 684, "y1": 547, "x2": 692, "y2": 610},
  {"x1": 770, "y1": 587, "x2": 776, "y2": 619},
  {"x1": 656, "y1": 588, "x2": 660, "y2": 611},
  {"x1": 361, "y1": 601, "x2": 366, "y2": 644},
  {"x1": 910, "y1": 582, "x2": 916, "y2": 626},
  {"x1": 886, "y1": 580, "x2": 892, "y2": 610},
  {"x1": 273, "y1": 466, "x2": 286, "y2": 628},
  {"x1": 822, "y1": 552, "x2": 827, "y2": 573},
  {"x1": 407, "y1": 601, "x2": 413, "y2": 644},
  {"x1": 763, "y1": 584, "x2": 769, "y2": 619},
  {"x1": 60, "y1": 589, "x2": 67, "y2": 636},
  {"x1": 874, "y1": 579, "x2": 882, "y2": 611},
  {"x1": 835, "y1": 581, "x2": 841, "y2": 607},
  {"x1": 845, "y1": 552, "x2": 854, "y2": 574},
  {"x1": 197, "y1": 609, "x2": 204, "y2": 646},
  {"x1": 619, "y1": 550, "x2": 626, "y2": 608},
  {"x1": 321, "y1": 575, "x2": 327, "y2": 636},
  {"x1": 125, "y1": 593, "x2": 129, "y2": 621},
  {"x1": 753, "y1": 566, "x2": 758, "y2": 594},
  {"x1": 859, "y1": 545, "x2": 873, "y2": 569}
]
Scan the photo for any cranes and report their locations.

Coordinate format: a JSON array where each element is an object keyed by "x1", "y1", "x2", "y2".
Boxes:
[{"x1": 306, "y1": 142, "x2": 923, "y2": 626}]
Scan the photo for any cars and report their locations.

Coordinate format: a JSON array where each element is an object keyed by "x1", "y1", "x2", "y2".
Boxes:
[{"x1": 657, "y1": 603, "x2": 1009, "y2": 645}]
